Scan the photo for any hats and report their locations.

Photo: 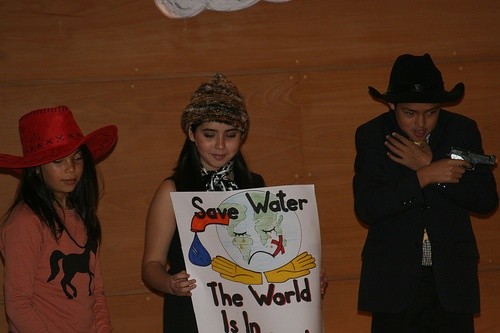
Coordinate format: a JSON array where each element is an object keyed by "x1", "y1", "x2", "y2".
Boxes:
[
  {"x1": 0, "y1": 104, "x2": 118, "y2": 179},
  {"x1": 181, "y1": 78, "x2": 250, "y2": 143},
  {"x1": 367, "y1": 52, "x2": 466, "y2": 104}
]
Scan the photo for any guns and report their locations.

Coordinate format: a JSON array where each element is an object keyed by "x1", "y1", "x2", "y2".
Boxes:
[{"x1": 433, "y1": 146, "x2": 497, "y2": 192}]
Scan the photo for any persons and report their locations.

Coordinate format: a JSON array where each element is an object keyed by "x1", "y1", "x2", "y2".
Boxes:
[
  {"x1": 0, "y1": 106, "x2": 120, "y2": 333},
  {"x1": 352, "y1": 54, "x2": 500, "y2": 333},
  {"x1": 142, "y1": 73, "x2": 328, "y2": 333}
]
